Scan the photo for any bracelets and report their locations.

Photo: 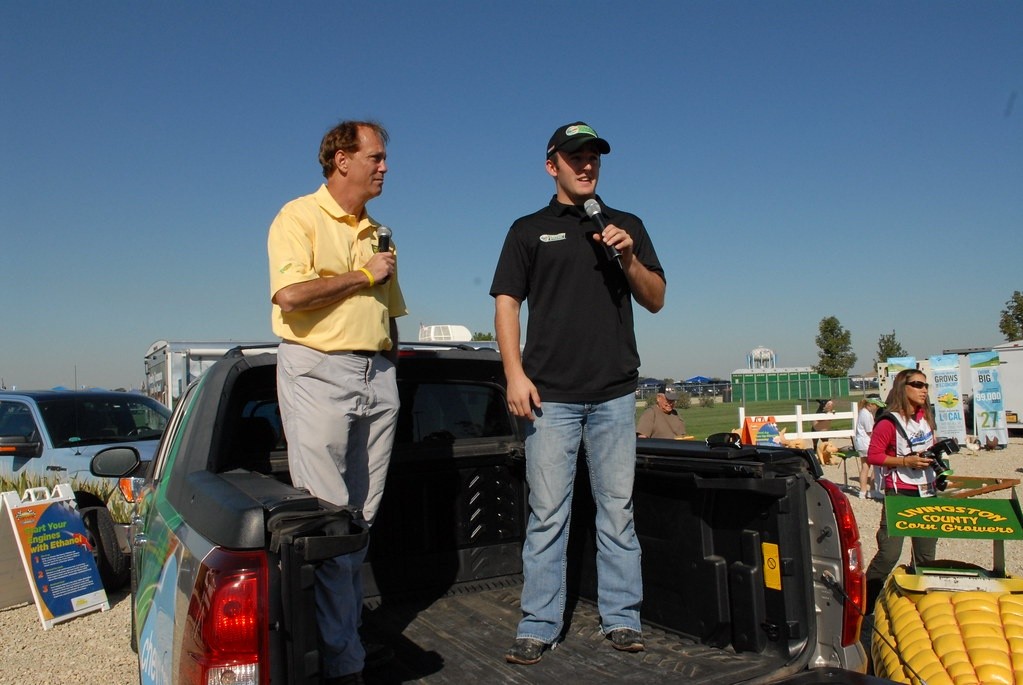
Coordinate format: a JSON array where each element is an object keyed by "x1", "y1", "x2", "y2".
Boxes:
[
  {"x1": 903, "y1": 456, "x2": 907, "y2": 468},
  {"x1": 358, "y1": 268, "x2": 374, "y2": 287}
]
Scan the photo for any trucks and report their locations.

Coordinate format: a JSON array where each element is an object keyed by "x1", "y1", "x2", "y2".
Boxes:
[{"x1": 144, "y1": 325, "x2": 526, "y2": 431}]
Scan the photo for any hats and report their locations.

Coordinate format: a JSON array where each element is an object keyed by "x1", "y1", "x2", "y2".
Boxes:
[
  {"x1": 866, "y1": 397, "x2": 887, "y2": 407},
  {"x1": 658, "y1": 383, "x2": 678, "y2": 401},
  {"x1": 547, "y1": 121, "x2": 611, "y2": 160}
]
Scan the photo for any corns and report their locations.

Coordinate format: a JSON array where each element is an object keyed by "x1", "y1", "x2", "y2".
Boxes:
[{"x1": 871, "y1": 561, "x2": 1023, "y2": 685}]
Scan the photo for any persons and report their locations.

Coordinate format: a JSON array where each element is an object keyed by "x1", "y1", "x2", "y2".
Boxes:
[
  {"x1": 812, "y1": 401, "x2": 835, "y2": 452},
  {"x1": 489, "y1": 122, "x2": 666, "y2": 663},
  {"x1": 266, "y1": 120, "x2": 408, "y2": 685},
  {"x1": 637, "y1": 384, "x2": 690, "y2": 441},
  {"x1": 860, "y1": 369, "x2": 959, "y2": 640},
  {"x1": 853, "y1": 393, "x2": 887, "y2": 500}
]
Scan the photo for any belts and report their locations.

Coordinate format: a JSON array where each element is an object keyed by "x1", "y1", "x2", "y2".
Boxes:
[{"x1": 353, "y1": 350, "x2": 377, "y2": 357}]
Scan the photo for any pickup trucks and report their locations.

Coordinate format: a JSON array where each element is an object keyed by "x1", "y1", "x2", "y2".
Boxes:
[{"x1": 90, "y1": 340, "x2": 907, "y2": 685}]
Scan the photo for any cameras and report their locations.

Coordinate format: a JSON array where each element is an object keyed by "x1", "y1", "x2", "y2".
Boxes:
[{"x1": 911, "y1": 438, "x2": 960, "y2": 491}]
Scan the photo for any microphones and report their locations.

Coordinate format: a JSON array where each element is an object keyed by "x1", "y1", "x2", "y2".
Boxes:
[
  {"x1": 584, "y1": 198, "x2": 622, "y2": 269},
  {"x1": 377, "y1": 226, "x2": 392, "y2": 284}
]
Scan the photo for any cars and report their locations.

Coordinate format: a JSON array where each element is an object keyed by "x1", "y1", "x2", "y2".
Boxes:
[
  {"x1": 636, "y1": 384, "x2": 720, "y2": 399},
  {"x1": 0, "y1": 387, "x2": 175, "y2": 594},
  {"x1": 850, "y1": 381, "x2": 878, "y2": 389}
]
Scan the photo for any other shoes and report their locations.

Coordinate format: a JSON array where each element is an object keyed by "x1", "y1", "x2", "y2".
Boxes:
[
  {"x1": 867, "y1": 491, "x2": 884, "y2": 499},
  {"x1": 859, "y1": 491, "x2": 867, "y2": 499},
  {"x1": 361, "y1": 641, "x2": 396, "y2": 668},
  {"x1": 612, "y1": 628, "x2": 647, "y2": 651},
  {"x1": 505, "y1": 638, "x2": 548, "y2": 664}
]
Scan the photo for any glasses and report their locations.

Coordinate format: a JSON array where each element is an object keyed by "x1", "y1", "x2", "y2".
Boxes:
[{"x1": 905, "y1": 380, "x2": 928, "y2": 390}]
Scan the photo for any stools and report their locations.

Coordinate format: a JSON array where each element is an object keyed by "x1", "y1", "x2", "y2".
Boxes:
[{"x1": 831, "y1": 451, "x2": 859, "y2": 492}]
